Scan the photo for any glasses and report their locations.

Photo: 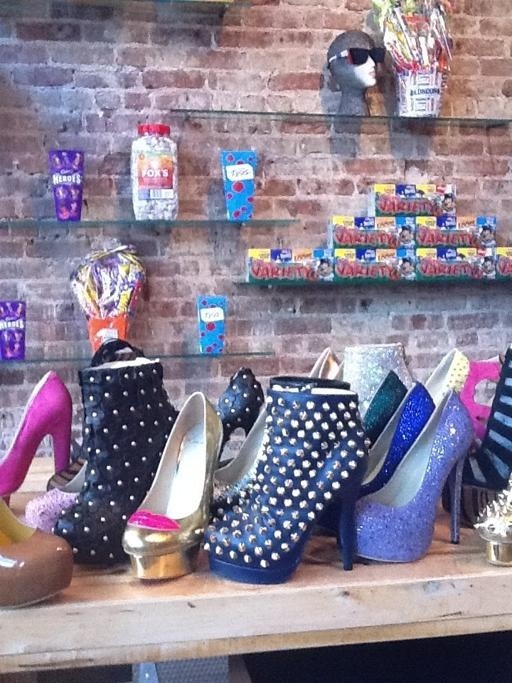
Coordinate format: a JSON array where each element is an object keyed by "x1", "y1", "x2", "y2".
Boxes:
[{"x1": 328, "y1": 46, "x2": 387, "y2": 66}]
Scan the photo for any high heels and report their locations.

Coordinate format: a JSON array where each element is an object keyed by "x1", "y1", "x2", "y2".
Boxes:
[
  {"x1": 25, "y1": 460, "x2": 88, "y2": 534},
  {"x1": 473, "y1": 472, "x2": 512, "y2": 567},
  {"x1": 356, "y1": 387, "x2": 476, "y2": 564},
  {"x1": 442, "y1": 347, "x2": 511, "y2": 528},
  {"x1": 210, "y1": 376, "x2": 351, "y2": 519},
  {"x1": 0, "y1": 495, "x2": 73, "y2": 609},
  {"x1": 203, "y1": 386, "x2": 371, "y2": 585},
  {"x1": 357, "y1": 380, "x2": 436, "y2": 499},
  {"x1": 424, "y1": 347, "x2": 469, "y2": 405},
  {"x1": 362, "y1": 370, "x2": 408, "y2": 449},
  {"x1": 122, "y1": 391, "x2": 223, "y2": 580},
  {"x1": 215, "y1": 366, "x2": 265, "y2": 467},
  {"x1": 0, "y1": 370, "x2": 73, "y2": 508},
  {"x1": 46, "y1": 336, "x2": 143, "y2": 493}
]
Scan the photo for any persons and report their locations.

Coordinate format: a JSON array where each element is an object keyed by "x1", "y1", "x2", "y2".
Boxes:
[{"x1": 327, "y1": 30, "x2": 377, "y2": 116}]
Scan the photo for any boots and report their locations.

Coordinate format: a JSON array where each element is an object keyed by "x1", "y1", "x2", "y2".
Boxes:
[{"x1": 54, "y1": 356, "x2": 181, "y2": 563}]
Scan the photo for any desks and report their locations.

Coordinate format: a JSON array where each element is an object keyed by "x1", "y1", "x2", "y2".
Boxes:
[{"x1": 0, "y1": 500, "x2": 512, "y2": 683}]
